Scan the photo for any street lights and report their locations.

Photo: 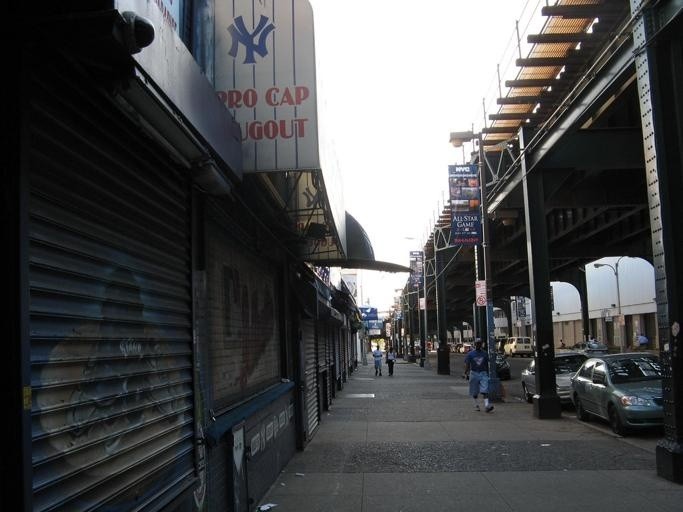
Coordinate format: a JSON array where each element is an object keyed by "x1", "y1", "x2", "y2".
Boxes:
[
  {"x1": 594, "y1": 256, "x2": 636, "y2": 353},
  {"x1": 448, "y1": 132, "x2": 503, "y2": 404},
  {"x1": 390, "y1": 250, "x2": 431, "y2": 370}
]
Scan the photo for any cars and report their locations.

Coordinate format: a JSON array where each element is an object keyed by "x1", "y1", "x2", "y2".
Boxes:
[
  {"x1": 463, "y1": 352, "x2": 512, "y2": 380},
  {"x1": 447, "y1": 344, "x2": 471, "y2": 354},
  {"x1": 522, "y1": 353, "x2": 665, "y2": 434}
]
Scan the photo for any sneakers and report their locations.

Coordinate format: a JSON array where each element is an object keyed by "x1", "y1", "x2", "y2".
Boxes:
[
  {"x1": 484, "y1": 405, "x2": 494, "y2": 412},
  {"x1": 473, "y1": 404, "x2": 480, "y2": 411}
]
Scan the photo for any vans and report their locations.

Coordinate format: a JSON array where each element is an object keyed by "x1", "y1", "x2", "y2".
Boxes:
[{"x1": 495, "y1": 337, "x2": 532, "y2": 358}]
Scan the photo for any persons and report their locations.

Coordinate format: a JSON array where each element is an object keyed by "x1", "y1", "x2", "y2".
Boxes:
[
  {"x1": 639, "y1": 332, "x2": 648, "y2": 345},
  {"x1": 464, "y1": 342, "x2": 494, "y2": 412},
  {"x1": 386, "y1": 347, "x2": 396, "y2": 376},
  {"x1": 559, "y1": 340, "x2": 563, "y2": 349},
  {"x1": 373, "y1": 346, "x2": 383, "y2": 376}
]
